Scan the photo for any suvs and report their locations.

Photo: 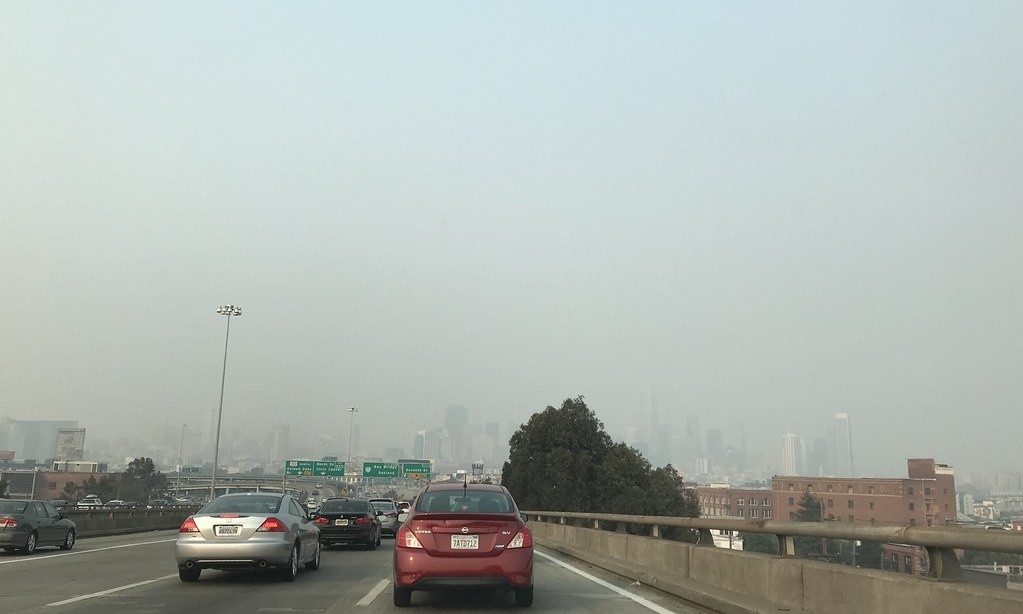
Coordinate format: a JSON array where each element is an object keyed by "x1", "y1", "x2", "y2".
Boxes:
[
  {"x1": 311, "y1": 489, "x2": 319, "y2": 495},
  {"x1": 76, "y1": 493, "x2": 102, "y2": 511},
  {"x1": 315, "y1": 483, "x2": 323, "y2": 488},
  {"x1": 307, "y1": 502, "x2": 317, "y2": 509},
  {"x1": 362, "y1": 498, "x2": 404, "y2": 537},
  {"x1": 395, "y1": 501, "x2": 411, "y2": 514},
  {"x1": 391, "y1": 482, "x2": 534, "y2": 607},
  {"x1": 307, "y1": 496, "x2": 315, "y2": 501},
  {"x1": 312, "y1": 497, "x2": 384, "y2": 551}
]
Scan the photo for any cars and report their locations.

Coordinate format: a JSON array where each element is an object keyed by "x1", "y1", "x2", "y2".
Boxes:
[
  {"x1": 174, "y1": 491, "x2": 322, "y2": 583},
  {"x1": 146, "y1": 499, "x2": 171, "y2": 510},
  {"x1": 102, "y1": 500, "x2": 127, "y2": 510},
  {"x1": 0, "y1": 499, "x2": 78, "y2": 555},
  {"x1": 49, "y1": 500, "x2": 75, "y2": 512}
]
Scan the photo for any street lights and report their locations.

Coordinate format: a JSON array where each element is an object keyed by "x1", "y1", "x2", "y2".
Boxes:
[
  {"x1": 347, "y1": 405, "x2": 357, "y2": 464},
  {"x1": 207, "y1": 301, "x2": 242, "y2": 505}
]
